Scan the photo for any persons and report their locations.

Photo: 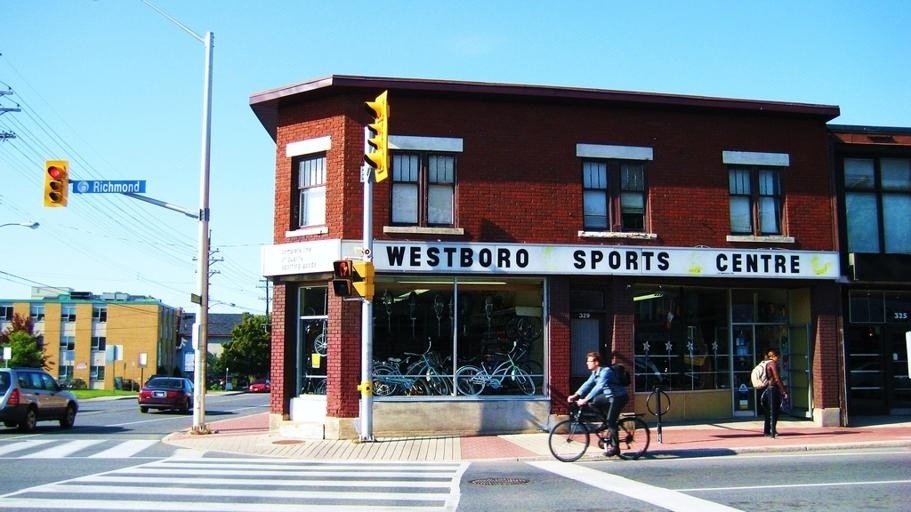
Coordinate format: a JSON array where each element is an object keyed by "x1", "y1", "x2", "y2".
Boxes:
[
  {"x1": 567, "y1": 352, "x2": 629, "y2": 456},
  {"x1": 761, "y1": 350, "x2": 789, "y2": 438}
]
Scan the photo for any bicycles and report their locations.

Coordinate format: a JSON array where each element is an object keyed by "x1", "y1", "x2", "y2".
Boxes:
[
  {"x1": 360, "y1": 307, "x2": 543, "y2": 401},
  {"x1": 549, "y1": 399, "x2": 651, "y2": 462},
  {"x1": 296, "y1": 310, "x2": 330, "y2": 395}
]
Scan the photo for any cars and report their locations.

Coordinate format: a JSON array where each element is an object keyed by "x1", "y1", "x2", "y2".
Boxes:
[
  {"x1": 138, "y1": 376, "x2": 195, "y2": 414},
  {"x1": 249, "y1": 378, "x2": 271, "y2": 393}
]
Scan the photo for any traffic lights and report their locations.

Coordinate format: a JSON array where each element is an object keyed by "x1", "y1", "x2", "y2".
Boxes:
[
  {"x1": 44, "y1": 160, "x2": 68, "y2": 208},
  {"x1": 333, "y1": 259, "x2": 352, "y2": 298},
  {"x1": 362, "y1": 91, "x2": 390, "y2": 183}
]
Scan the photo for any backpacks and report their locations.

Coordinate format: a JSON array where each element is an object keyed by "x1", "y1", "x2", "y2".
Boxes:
[
  {"x1": 750, "y1": 360, "x2": 772, "y2": 389},
  {"x1": 595, "y1": 365, "x2": 631, "y2": 386}
]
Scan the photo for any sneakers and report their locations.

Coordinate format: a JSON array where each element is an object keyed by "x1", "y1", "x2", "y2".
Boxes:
[
  {"x1": 598, "y1": 423, "x2": 608, "y2": 430},
  {"x1": 764, "y1": 432, "x2": 778, "y2": 438},
  {"x1": 604, "y1": 447, "x2": 619, "y2": 456}
]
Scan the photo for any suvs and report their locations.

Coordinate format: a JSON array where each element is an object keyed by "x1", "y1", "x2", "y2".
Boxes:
[{"x1": 1, "y1": 367, "x2": 80, "y2": 432}]
